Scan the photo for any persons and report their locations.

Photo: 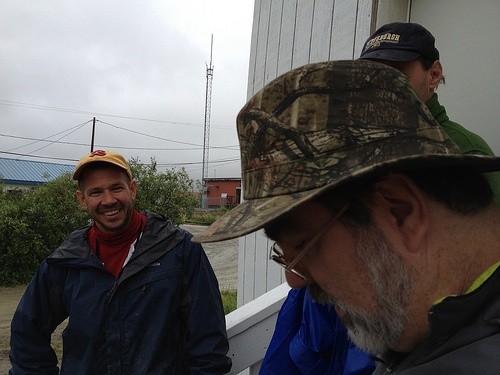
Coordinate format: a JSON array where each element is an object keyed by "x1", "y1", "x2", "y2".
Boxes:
[
  {"x1": 190, "y1": 21, "x2": 500, "y2": 375},
  {"x1": 9, "y1": 149, "x2": 233, "y2": 375}
]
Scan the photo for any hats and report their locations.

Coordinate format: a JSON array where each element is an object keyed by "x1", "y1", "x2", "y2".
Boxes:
[
  {"x1": 73, "y1": 149, "x2": 133, "y2": 180},
  {"x1": 357, "y1": 22, "x2": 439, "y2": 59},
  {"x1": 191, "y1": 59, "x2": 500, "y2": 243}
]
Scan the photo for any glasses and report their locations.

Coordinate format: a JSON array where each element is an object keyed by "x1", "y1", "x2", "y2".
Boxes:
[{"x1": 269, "y1": 195, "x2": 359, "y2": 280}]
